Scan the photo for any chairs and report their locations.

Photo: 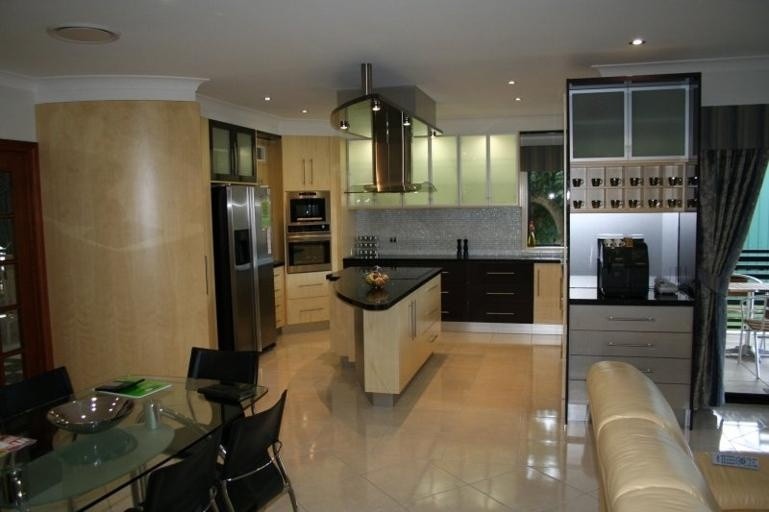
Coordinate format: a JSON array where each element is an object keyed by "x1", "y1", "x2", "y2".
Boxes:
[{"x1": 737, "y1": 296, "x2": 769, "y2": 380}]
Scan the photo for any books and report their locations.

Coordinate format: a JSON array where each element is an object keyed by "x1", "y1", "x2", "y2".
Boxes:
[
  {"x1": 0, "y1": 433, "x2": 37, "y2": 459},
  {"x1": 92, "y1": 376, "x2": 173, "y2": 400}
]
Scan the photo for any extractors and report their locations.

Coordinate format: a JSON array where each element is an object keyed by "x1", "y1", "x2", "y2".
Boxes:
[{"x1": 327, "y1": 92, "x2": 446, "y2": 195}]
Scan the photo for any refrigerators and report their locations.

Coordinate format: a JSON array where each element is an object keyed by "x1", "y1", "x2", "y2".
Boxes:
[{"x1": 210, "y1": 183, "x2": 278, "y2": 355}]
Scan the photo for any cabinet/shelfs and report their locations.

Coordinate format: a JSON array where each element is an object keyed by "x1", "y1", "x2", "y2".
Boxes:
[
  {"x1": 567, "y1": 302, "x2": 694, "y2": 435},
  {"x1": 362, "y1": 276, "x2": 443, "y2": 395},
  {"x1": 418, "y1": 259, "x2": 533, "y2": 325},
  {"x1": 283, "y1": 272, "x2": 336, "y2": 324},
  {"x1": 532, "y1": 263, "x2": 565, "y2": 324},
  {"x1": 563, "y1": 82, "x2": 699, "y2": 212},
  {"x1": 208, "y1": 120, "x2": 258, "y2": 185}
]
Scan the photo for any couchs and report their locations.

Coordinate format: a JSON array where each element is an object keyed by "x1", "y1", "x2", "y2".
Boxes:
[{"x1": 586, "y1": 359, "x2": 769, "y2": 510}]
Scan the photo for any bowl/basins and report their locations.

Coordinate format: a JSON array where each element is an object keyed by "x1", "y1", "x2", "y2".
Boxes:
[
  {"x1": 361, "y1": 271, "x2": 393, "y2": 290},
  {"x1": 46, "y1": 395, "x2": 135, "y2": 435}
]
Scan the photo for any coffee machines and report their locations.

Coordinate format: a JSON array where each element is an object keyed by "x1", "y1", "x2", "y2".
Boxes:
[{"x1": 596, "y1": 237, "x2": 650, "y2": 298}]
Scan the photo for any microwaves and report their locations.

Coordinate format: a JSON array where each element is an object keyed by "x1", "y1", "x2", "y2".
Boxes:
[{"x1": 286, "y1": 189, "x2": 332, "y2": 235}]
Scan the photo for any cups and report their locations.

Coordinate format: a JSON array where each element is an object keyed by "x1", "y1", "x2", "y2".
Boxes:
[
  {"x1": 610, "y1": 177, "x2": 622, "y2": 186},
  {"x1": 604, "y1": 237, "x2": 634, "y2": 249},
  {"x1": 572, "y1": 178, "x2": 584, "y2": 187},
  {"x1": 592, "y1": 199, "x2": 603, "y2": 208},
  {"x1": 628, "y1": 199, "x2": 640, "y2": 208},
  {"x1": 667, "y1": 199, "x2": 677, "y2": 208},
  {"x1": 690, "y1": 176, "x2": 699, "y2": 185},
  {"x1": 591, "y1": 178, "x2": 603, "y2": 187},
  {"x1": 668, "y1": 177, "x2": 680, "y2": 186},
  {"x1": 687, "y1": 199, "x2": 696, "y2": 207},
  {"x1": 610, "y1": 199, "x2": 622, "y2": 208},
  {"x1": 573, "y1": 200, "x2": 585, "y2": 209},
  {"x1": 648, "y1": 199, "x2": 659, "y2": 208},
  {"x1": 650, "y1": 176, "x2": 661, "y2": 186},
  {"x1": 630, "y1": 177, "x2": 640, "y2": 186}
]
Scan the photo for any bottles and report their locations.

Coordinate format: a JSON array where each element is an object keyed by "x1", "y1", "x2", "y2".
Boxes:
[
  {"x1": 456, "y1": 238, "x2": 463, "y2": 258},
  {"x1": 463, "y1": 238, "x2": 469, "y2": 258}
]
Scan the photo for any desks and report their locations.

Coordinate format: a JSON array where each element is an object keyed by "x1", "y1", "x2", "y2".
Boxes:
[{"x1": 725, "y1": 281, "x2": 769, "y2": 360}]
